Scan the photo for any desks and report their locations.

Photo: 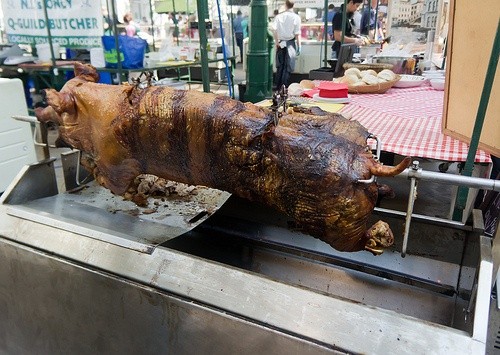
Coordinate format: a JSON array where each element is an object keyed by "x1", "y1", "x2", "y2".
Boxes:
[{"x1": 286, "y1": 77, "x2": 494, "y2": 226}]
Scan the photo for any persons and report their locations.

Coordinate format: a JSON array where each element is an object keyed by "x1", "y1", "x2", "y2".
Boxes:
[
  {"x1": 332, "y1": 0, "x2": 375, "y2": 60},
  {"x1": 224, "y1": 10, "x2": 250, "y2": 63},
  {"x1": 352, "y1": 0, "x2": 389, "y2": 53},
  {"x1": 321, "y1": 4, "x2": 337, "y2": 40},
  {"x1": 266, "y1": 0, "x2": 302, "y2": 93},
  {"x1": 104, "y1": 11, "x2": 196, "y2": 66}
]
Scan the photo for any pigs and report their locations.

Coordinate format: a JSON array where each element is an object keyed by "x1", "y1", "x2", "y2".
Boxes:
[{"x1": 34, "y1": 57, "x2": 414, "y2": 258}]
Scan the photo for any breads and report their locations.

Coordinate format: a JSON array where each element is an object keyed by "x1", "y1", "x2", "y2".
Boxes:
[
  {"x1": 288, "y1": 83, "x2": 303, "y2": 94},
  {"x1": 334, "y1": 67, "x2": 396, "y2": 85},
  {"x1": 300, "y1": 80, "x2": 315, "y2": 89}
]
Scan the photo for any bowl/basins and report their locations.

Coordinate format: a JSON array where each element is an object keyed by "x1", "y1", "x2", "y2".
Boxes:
[
  {"x1": 335, "y1": 73, "x2": 401, "y2": 94},
  {"x1": 393, "y1": 74, "x2": 427, "y2": 87},
  {"x1": 423, "y1": 70, "x2": 446, "y2": 90}
]
